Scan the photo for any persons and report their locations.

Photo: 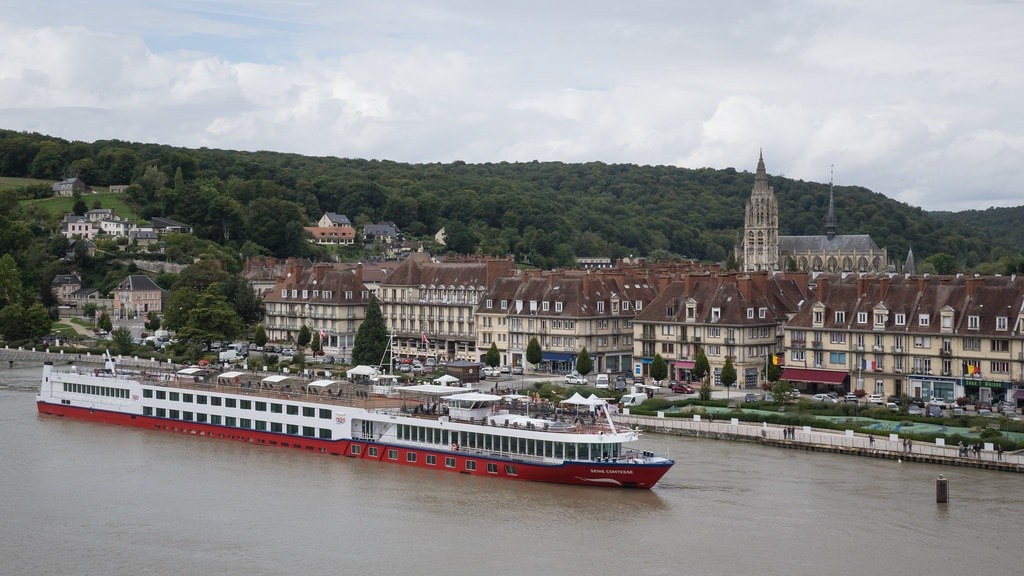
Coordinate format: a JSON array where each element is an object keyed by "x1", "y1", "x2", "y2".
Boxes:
[
  {"x1": 868, "y1": 434, "x2": 875, "y2": 446},
  {"x1": 956, "y1": 440, "x2": 1005, "y2": 461},
  {"x1": 782, "y1": 425, "x2": 795, "y2": 439},
  {"x1": 902, "y1": 436, "x2": 913, "y2": 452}
]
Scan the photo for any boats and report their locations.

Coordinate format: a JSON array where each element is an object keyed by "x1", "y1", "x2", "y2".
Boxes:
[{"x1": 34, "y1": 358, "x2": 678, "y2": 491}]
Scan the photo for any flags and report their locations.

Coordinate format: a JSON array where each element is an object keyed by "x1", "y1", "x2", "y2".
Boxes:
[
  {"x1": 320, "y1": 329, "x2": 326, "y2": 338},
  {"x1": 422, "y1": 335, "x2": 429, "y2": 343}
]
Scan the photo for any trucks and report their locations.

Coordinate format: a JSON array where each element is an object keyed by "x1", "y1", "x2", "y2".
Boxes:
[{"x1": 618, "y1": 393, "x2": 647, "y2": 408}]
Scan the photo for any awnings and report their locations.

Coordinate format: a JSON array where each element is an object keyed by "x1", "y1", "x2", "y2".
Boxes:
[
  {"x1": 779, "y1": 368, "x2": 849, "y2": 385},
  {"x1": 674, "y1": 361, "x2": 696, "y2": 369},
  {"x1": 1013, "y1": 389, "x2": 1024, "y2": 399},
  {"x1": 541, "y1": 352, "x2": 572, "y2": 362}
]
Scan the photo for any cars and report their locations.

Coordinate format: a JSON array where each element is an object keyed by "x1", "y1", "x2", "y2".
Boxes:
[
  {"x1": 887, "y1": 395, "x2": 901, "y2": 406},
  {"x1": 949, "y1": 409, "x2": 967, "y2": 417},
  {"x1": 926, "y1": 404, "x2": 944, "y2": 418},
  {"x1": 912, "y1": 397, "x2": 924, "y2": 408},
  {"x1": 950, "y1": 399, "x2": 967, "y2": 411},
  {"x1": 672, "y1": 383, "x2": 695, "y2": 394},
  {"x1": 787, "y1": 388, "x2": 800, "y2": 399},
  {"x1": 885, "y1": 403, "x2": 899, "y2": 412},
  {"x1": 745, "y1": 393, "x2": 758, "y2": 403},
  {"x1": 844, "y1": 392, "x2": 858, "y2": 403},
  {"x1": 813, "y1": 394, "x2": 841, "y2": 404},
  {"x1": 976, "y1": 409, "x2": 994, "y2": 417},
  {"x1": 909, "y1": 404, "x2": 922, "y2": 417},
  {"x1": 1002, "y1": 410, "x2": 1021, "y2": 422},
  {"x1": 91, "y1": 326, "x2": 678, "y2": 394},
  {"x1": 997, "y1": 401, "x2": 1016, "y2": 412},
  {"x1": 866, "y1": 395, "x2": 883, "y2": 405},
  {"x1": 975, "y1": 402, "x2": 992, "y2": 412},
  {"x1": 929, "y1": 397, "x2": 947, "y2": 409}
]
